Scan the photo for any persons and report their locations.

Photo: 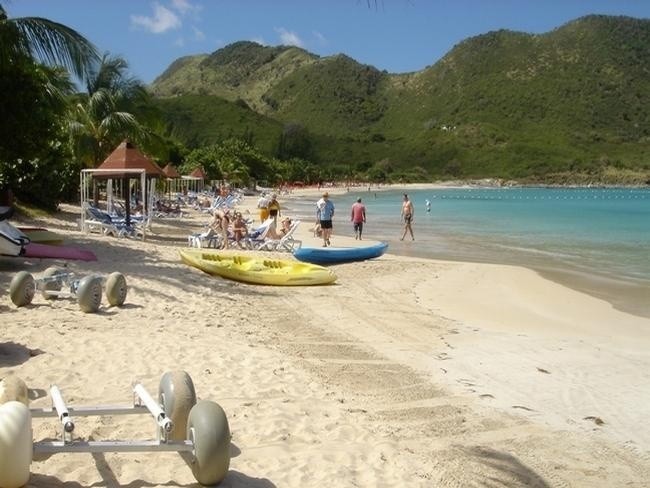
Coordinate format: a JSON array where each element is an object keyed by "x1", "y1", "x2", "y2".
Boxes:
[
  {"x1": 426, "y1": 199, "x2": 432, "y2": 214},
  {"x1": 118, "y1": 171, "x2": 378, "y2": 250},
  {"x1": 399, "y1": 193, "x2": 415, "y2": 242}
]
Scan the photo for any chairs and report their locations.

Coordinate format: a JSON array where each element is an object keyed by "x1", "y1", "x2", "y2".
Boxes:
[{"x1": 82, "y1": 187, "x2": 301, "y2": 252}]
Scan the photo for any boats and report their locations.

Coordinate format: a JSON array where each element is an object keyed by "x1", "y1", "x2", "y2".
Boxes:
[
  {"x1": 0, "y1": 220, "x2": 29, "y2": 257},
  {"x1": 291, "y1": 241, "x2": 389, "y2": 265},
  {"x1": 177, "y1": 248, "x2": 338, "y2": 285}
]
